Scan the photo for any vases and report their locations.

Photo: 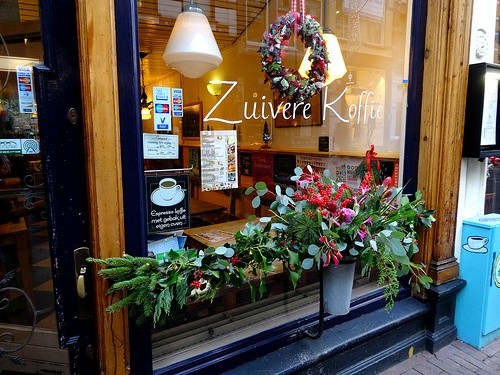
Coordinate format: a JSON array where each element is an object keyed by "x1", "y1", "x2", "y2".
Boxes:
[{"x1": 323, "y1": 255, "x2": 358, "y2": 315}]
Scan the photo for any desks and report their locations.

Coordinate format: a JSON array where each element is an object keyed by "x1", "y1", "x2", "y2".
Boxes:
[
  {"x1": 184, "y1": 197, "x2": 266, "y2": 248},
  {"x1": 0, "y1": 217, "x2": 39, "y2": 316}
]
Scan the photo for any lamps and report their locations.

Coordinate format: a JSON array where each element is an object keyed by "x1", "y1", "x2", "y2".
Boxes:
[
  {"x1": 160, "y1": 0, "x2": 223, "y2": 79},
  {"x1": 207, "y1": 84, "x2": 222, "y2": 96},
  {"x1": 139, "y1": 51, "x2": 151, "y2": 120}
]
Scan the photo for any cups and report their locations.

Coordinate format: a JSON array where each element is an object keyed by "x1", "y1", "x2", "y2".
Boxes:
[{"x1": 159, "y1": 178, "x2": 181, "y2": 200}]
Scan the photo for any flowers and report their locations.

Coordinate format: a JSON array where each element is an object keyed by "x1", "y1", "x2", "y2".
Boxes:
[
  {"x1": 258, "y1": 12, "x2": 329, "y2": 100},
  {"x1": 247, "y1": 165, "x2": 437, "y2": 312}
]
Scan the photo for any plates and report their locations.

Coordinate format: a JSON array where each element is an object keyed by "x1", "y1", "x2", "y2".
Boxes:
[{"x1": 151, "y1": 188, "x2": 185, "y2": 206}]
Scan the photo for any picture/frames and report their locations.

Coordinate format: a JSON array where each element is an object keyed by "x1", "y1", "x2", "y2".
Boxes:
[{"x1": 274, "y1": 84, "x2": 323, "y2": 127}]
